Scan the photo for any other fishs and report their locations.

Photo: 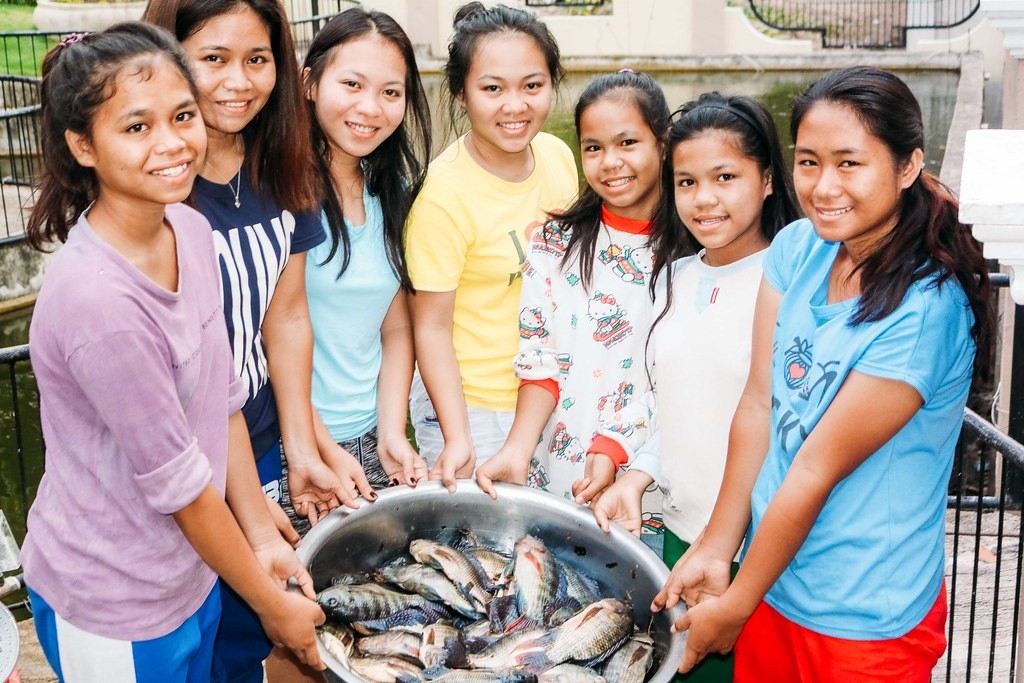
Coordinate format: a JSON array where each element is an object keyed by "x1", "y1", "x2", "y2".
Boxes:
[{"x1": 314, "y1": 523, "x2": 655, "y2": 683}]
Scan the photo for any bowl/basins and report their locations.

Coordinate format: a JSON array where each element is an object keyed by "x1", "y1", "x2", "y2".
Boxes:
[{"x1": 287, "y1": 479, "x2": 688, "y2": 683}]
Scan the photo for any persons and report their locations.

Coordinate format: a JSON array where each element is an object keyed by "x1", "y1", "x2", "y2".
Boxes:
[
  {"x1": 18, "y1": 22, "x2": 327, "y2": 683},
  {"x1": 474, "y1": 68, "x2": 673, "y2": 561},
  {"x1": 597, "y1": 94, "x2": 798, "y2": 683},
  {"x1": 664, "y1": 67, "x2": 995, "y2": 683},
  {"x1": 280, "y1": 6, "x2": 433, "y2": 541},
  {"x1": 403, "y1": 0, "x2": 578, "y2": 494},
  {"x1": 140, "y1": 0, "x2": 360, "y2": 683}
]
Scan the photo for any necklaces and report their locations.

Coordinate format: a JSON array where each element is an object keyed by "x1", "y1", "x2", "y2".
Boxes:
[{"x1": 204, "y1": 135, "x2": 246, "y2": 208}]
